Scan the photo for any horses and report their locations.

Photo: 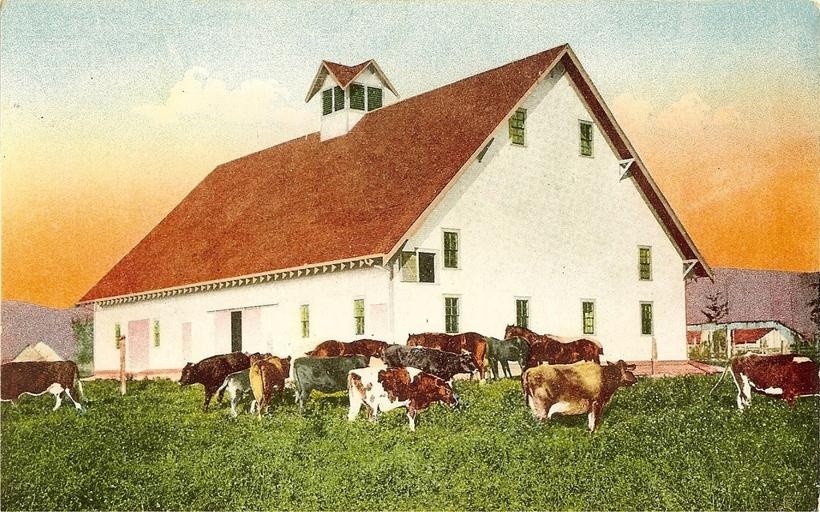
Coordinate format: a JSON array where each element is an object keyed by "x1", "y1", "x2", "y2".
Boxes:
[
  {"x1": 306, "y1": 338, "x2": 387, "y2": 358},
  {"x1": 488, "y1": 324, "x2": 604, "y2": 385}
]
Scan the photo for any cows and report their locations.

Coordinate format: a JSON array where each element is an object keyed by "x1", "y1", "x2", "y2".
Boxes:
[
  {"x1": 0, "y1": 360, "x2": 89, "y2": 411},
  {"x1": 384, "y1": 343, "x2": 478, "y2": 389},
  {"x1": 346, "y1": 366, "x2": 459, "y2": 432},
  {"x1": 289, "y1": 354, "x2": 368, "y2": 415},
  {"x1": 710, "y1": 352, "x2": 820, "y2": 409},
  {"x1": 520, "y1": 358, "x2": 638, "y2": 433},
  {"x1": 406, "y1": 331, "x2": 487, "y2": 385},
  {"x1": 179, "y1": 350, "x2": 292, "y2": 421}
]
